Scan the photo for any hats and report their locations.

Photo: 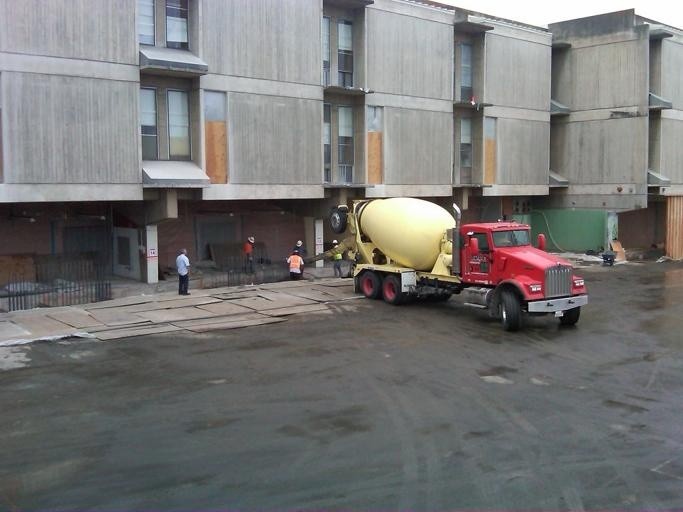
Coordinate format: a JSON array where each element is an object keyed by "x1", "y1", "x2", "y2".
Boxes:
[
  {"x1": 332, "y1": 240, "x2": 338, "y2": 243},
  {"x1": 247, "y1": 237, "x2": 255, "y2": 243}
]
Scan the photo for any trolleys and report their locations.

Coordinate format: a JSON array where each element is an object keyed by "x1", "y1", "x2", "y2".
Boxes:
[{"x1": 601, "y1": 250, "x2": 619, "y2": 266}]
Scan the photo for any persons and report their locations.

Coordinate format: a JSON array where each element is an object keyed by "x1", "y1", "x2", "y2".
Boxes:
[
  {"x1": 174, "y1": 248, "x2": 192, "y2": 296},
  {"x1": 330, "y1": 239, "x2": 343, "y2": 278},
  {"x1": 291, "y1": 239, "x2": 305, "y2": 278},
  {"x1": 243, "y1": 236, "x2": 257, "y2": 273},
  {"x1": 284, "y1": 249, "x2": 304, "y2": 281}
]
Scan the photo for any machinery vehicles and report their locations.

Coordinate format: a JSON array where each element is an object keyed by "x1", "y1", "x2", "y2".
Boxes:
[{"x1": 329, "y1": 194, "x2": 589, "y2": 333}]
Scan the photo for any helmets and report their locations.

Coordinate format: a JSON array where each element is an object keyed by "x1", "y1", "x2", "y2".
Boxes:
[{"x1": 296, "y1": 240, "x2": 303, "y2": 247}]
